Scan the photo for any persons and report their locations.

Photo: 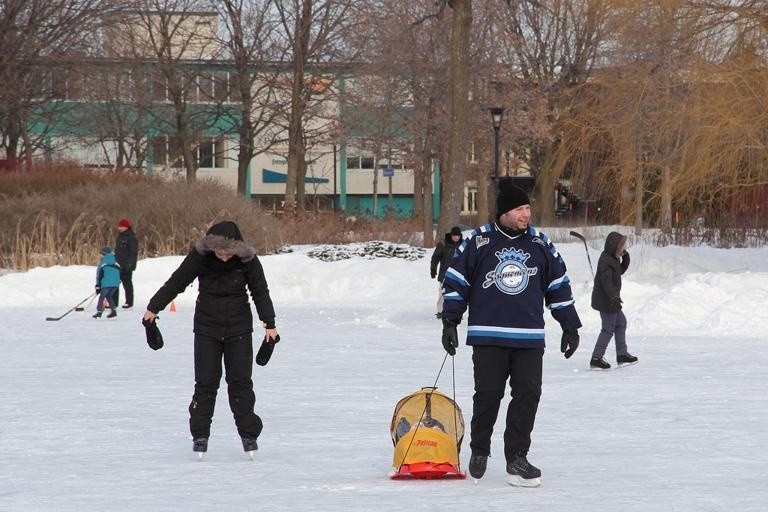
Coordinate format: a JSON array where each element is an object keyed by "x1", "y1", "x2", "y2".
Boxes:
[
  {"x1": 103, "y1": 219, "x2": 138, "y2": 310},
  {"x1": 92, "y1": 244, "x2": 123, "y2": 318},
  {"x1": 141, "y1": 219, "x2": 279, "y2": 454},
  {"x1": 438, "y1": 177, "x2": 583, "y2": 479},
  {"x1": 590, "y1": 230, "x2": 638, "y2": 370},
  {"x1": 428, "y1": 224, "x2": 466, "y2": 319}
]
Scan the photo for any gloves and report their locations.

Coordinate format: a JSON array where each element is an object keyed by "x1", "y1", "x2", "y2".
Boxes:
[
  {"x1": 142, "y1": 317, "x2": 163, "y2": 350},
  {"x1": 561, "y1": 331, "x2": 579, "y2": 358},
  {"x1": 442, "y1": 323, "x2": 458, "y2": 356},
  {"x1": 256, "y1": 335, "x2": 280, "y2": 366},
  {"x1": 96, "y1": 285, "x2": 101, "y2": 294}
]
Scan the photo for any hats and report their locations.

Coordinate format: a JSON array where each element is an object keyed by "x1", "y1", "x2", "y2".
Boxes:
[
  {"x1": 451, "y1": 227, "x2": 461, "y2": 236},
  {"x1": 101, "y1": 246, "x2": 111, "y2": 255},
  {"x1": 119, "y1": 219, "x2": 129, "y2": 227},
  {"x1": 498, "y1": 185, "x2": 530, "y2": 213}
]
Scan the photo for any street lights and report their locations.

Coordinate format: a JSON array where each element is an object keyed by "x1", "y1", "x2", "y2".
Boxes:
[{"x1": 484, "y1": 104, "x2": 508, "y2": 178}]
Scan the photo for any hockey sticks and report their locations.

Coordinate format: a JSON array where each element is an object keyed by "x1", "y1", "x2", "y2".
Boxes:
[
  {"x1": 45, "y1": 290, "x2": 97, "y2": 322},
  {"x1": 569, "y1": 230, "x2": 595, "y2": 276}
]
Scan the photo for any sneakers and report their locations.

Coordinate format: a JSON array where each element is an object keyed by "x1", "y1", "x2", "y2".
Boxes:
[
  {"x1": 93, "y1": 303, "x2": 130, "y2": 317},
  {"x1": 590, "y1": 358, "x2": 610, "y2": 368},
  {"x1": 193, "y1": 440, "x2": 207, "y2": 452},
  {"x1": 242, "y1": 440, "x2": 258, "y2": 450},
  {"x1": 507, "y1": 458, "x2": 540, "y2": 479},
  {"x1": 617, "y1": 353, "x2": 637, "y2": 362},
  {"x1": 469, "y1": 455, "x2": 487, "y2": 478}
]
